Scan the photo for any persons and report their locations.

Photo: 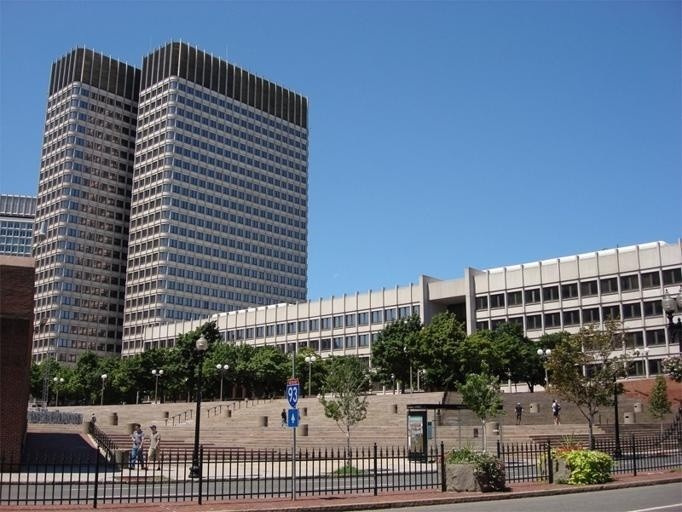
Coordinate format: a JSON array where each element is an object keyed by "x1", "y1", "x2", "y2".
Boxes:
[
  {"x1": 515, "y1": 402, "x2": 523, "y2": 425},
  {"x1": 128, "y1": 424, "x2": 161, "y2": 471},
  {"x1": 281, "y1": 408, "x2": 287, "y2": 432},
  {"x1": 552, "y1": 399, "x2": 561, "y2": 426}
]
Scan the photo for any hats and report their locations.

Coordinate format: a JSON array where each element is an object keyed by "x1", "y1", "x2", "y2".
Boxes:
[{"x1": 150, "y1": 425, "x2": 157, "y2": 428}]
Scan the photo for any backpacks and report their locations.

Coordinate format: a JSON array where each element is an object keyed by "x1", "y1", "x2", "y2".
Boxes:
[{"x1": 555, "y1": 404, "x2": 561, "y2": 413}]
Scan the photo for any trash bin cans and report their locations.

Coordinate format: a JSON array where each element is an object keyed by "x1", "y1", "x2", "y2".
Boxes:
[
  {"x1": 624, "y1": 412, "x2": 635, "y2": 424},
  {"x1": 529, "y1": 403, "x2": 539, "y2": 414},
  {"x1": 427, "y1": 421, "x2": 432, "y2": 439},
  {"x1": 392, "y1": 404, "x2": 397, "y2": 414},
  {"x1": 260, "y1": 416, "x2": 268, "y2": 427},
  {"x1": 300, "y1": 407, "x2": 307, "y2": 416},
  {"x1": 486, "y1": 422, "x2": 500, "y2": 435},
  {"x1": 109, "y1": 412, "x2": 118, "y2": 425},
  {"x1": 163, "y1": 411, "x2": 169, "y2": 418},
  {"x1": 634, "y1": 403, "x2": 643, "y2": 412},
  {"x1": 224, "y1": 410, "x2": 231, "y2": 417},
  {"x1": 297, "y1": 424, "x2": 308, "y2": 436},
  {"x1": 116, "y1": 450, "x2": 129, "y2": 469}
]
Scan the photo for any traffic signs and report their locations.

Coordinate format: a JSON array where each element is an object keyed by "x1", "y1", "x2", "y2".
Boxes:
[{"x1": 286, "y1": 377, "x2": 299, "y2": 407}]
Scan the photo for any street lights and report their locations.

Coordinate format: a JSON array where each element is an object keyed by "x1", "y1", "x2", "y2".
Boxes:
[
  {"x1": 215, "y1": 364, "x2": 229, "y2": 403},
  {"x1": 100, "y1": 374, "x2": 108, "y2": 405},
  {"x1": 185, "y1": 331, "x2": 208, "y2": 478},
  {"x1": 52, "y1": 376, "x2": 65, "y2": 407},
  {"x1": 535, "y1": 348, "x2": 556, "y2": 392},
  {"x1": 661, "y1": 282, "x2": 682, "y2": 353},
  {"x1": 151, "y1": 369, "x2": 164, "y2": 405},
  {"x1": 303, "y1": 355, "x2": 317, "y2": 397}
]
